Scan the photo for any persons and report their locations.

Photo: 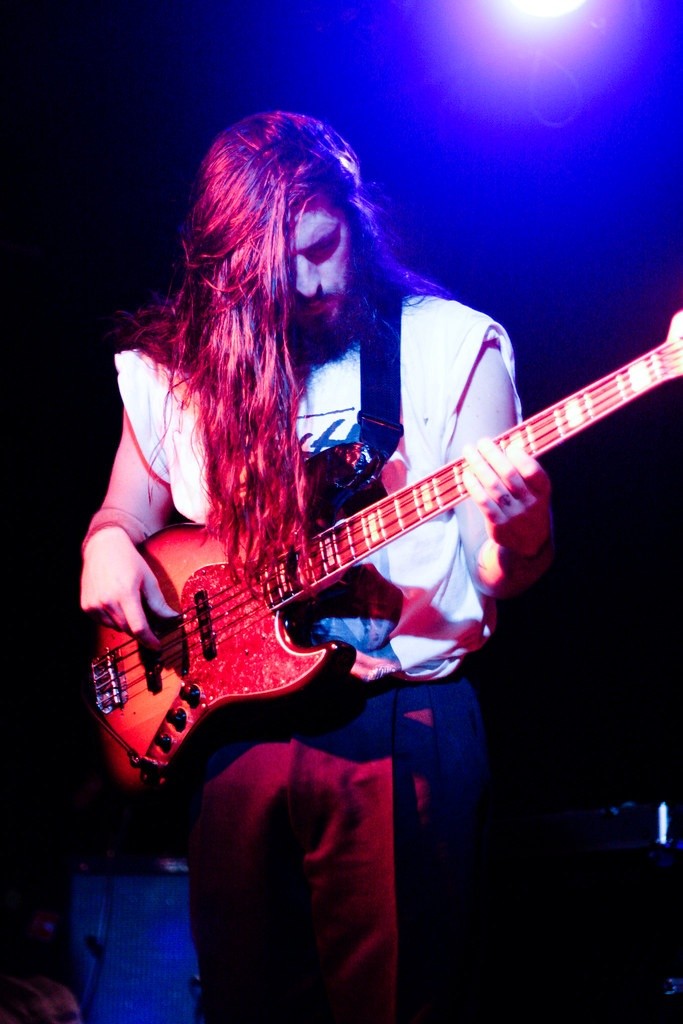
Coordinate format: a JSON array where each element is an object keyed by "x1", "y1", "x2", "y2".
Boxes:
[{"x1": 79, "y1": 112, "x2": 554, "y2": 1024}]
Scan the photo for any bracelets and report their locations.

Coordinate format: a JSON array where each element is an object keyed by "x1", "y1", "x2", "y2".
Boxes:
[{"x1": 82, "y1": 521, "x2": 132, "y2": 550}]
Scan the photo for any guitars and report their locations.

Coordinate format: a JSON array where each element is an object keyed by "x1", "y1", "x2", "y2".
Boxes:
[{"x1": 86, "y1": 307, "x2": 683, "y2": 795}]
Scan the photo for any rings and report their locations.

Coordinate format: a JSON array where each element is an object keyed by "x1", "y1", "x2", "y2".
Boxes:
[{"x1": 497, "y1": 493, "x2": 513, "y2": 507}]
